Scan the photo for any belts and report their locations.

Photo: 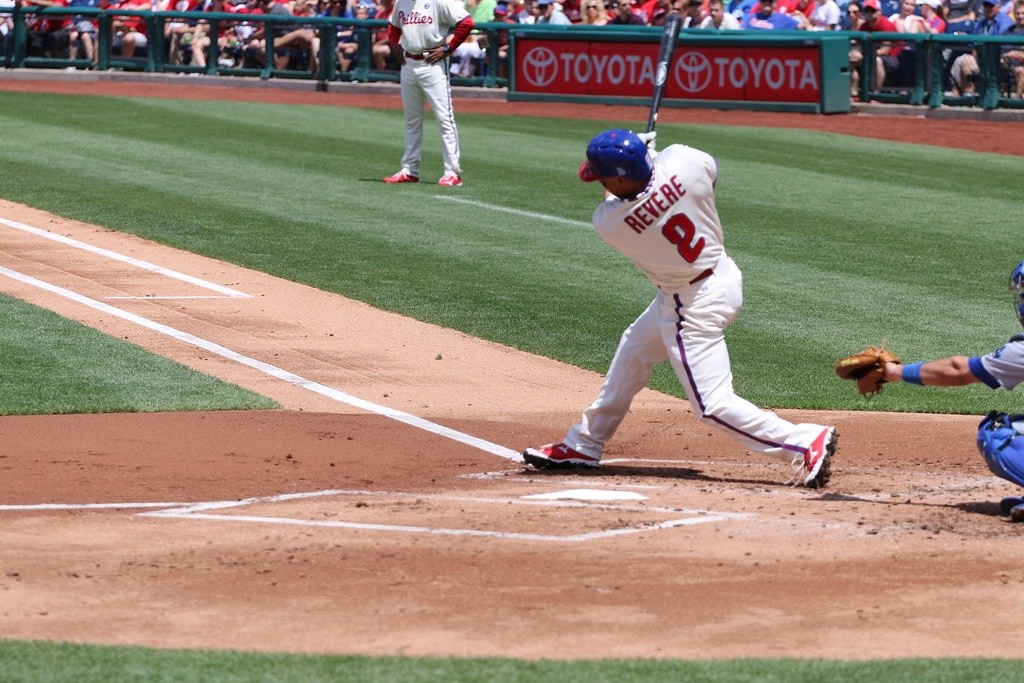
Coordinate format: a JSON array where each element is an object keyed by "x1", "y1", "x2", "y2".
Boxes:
[
  {"x1": 405, "y1": 51, "x2": 433, "y2": 60},
  {"x1": 687, "y1": 267, "x2": 713, "y2": 285}
]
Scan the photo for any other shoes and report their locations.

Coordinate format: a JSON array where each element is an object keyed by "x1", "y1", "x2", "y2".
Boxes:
[{"x1": 1001, "y1": 497, "x2": 1024, "y2": 519}]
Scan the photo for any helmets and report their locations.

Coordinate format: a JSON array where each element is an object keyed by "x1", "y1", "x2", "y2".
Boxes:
[{"x1": 577, "y1": 130, "x2": 651, "y2": 183}]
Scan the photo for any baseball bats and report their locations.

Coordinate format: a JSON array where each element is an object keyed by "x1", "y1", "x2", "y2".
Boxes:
[{"x1": 644, "y1": 12, "x2": 685, "y2": 150}]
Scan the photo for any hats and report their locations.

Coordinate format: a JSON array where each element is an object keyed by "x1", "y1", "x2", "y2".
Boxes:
[
  {"x1": 983, "y1": 0, "x2": 1000, "y2": 5},
  {"x1": 494, "y1": 5, "x2": 508, "y2": 16},
  {"x1": 862, "y1": 0, "x2": 881, "y2": 10},
  {"x1": 538, "y1": 0, "x2": 553, "y2": 8}
]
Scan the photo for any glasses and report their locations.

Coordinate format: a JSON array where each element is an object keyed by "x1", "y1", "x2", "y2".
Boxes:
[
  {"x1": 586, "y1": 5, "x2": 598, "y2": 9},
  {"x1": 690, "y1": 2, "x2": 703, "y2": 7},
  {"x1": 673, "y1": 8, "x2": 682, "y2": 11},
  {"x1": 605, "y1": 5, "x2": 611, "y2": 9}
]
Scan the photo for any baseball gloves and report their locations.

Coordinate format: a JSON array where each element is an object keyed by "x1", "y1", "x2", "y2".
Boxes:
[{"x1": 835, "y1": 338, "x2": 902, "y2": 406}]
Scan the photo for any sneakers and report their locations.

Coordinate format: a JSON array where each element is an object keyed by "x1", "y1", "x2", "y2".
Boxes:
[
  {"x1": 524, "y1": 442, "x2": 599, "y2": 469},
  {"x1": 785, "y1": 427, "x2": 839, "y2": 491},
  {"x1": 384, "y1": 170, "x2": 419, "y2": 183},
  {"x1": 439, "y1": 172, "x2": 462, "y2": 189}
]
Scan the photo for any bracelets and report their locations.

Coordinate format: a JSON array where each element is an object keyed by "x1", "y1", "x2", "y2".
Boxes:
[{"x1": 902, "y1": 360, "x2": 927, "y2": 385}]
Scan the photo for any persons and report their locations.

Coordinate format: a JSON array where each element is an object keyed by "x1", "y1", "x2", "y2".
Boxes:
[
  {"x1": 384, "y1": 0, "x2": 475, "y2": 185},
  {"x1": 524, "y1": 130, "x2": 840, "y2": 489},
  {"x1": 0, "y1": 0, "x2": 1024, "y2": 104},
  {"x1": 837, "y1": 260, "x2": 1024, "y2": 523}
]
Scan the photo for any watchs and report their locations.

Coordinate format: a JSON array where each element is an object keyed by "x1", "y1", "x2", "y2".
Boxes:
[{"x1": 443, "y1": 45, "x2": 451, "y2": 56}]
